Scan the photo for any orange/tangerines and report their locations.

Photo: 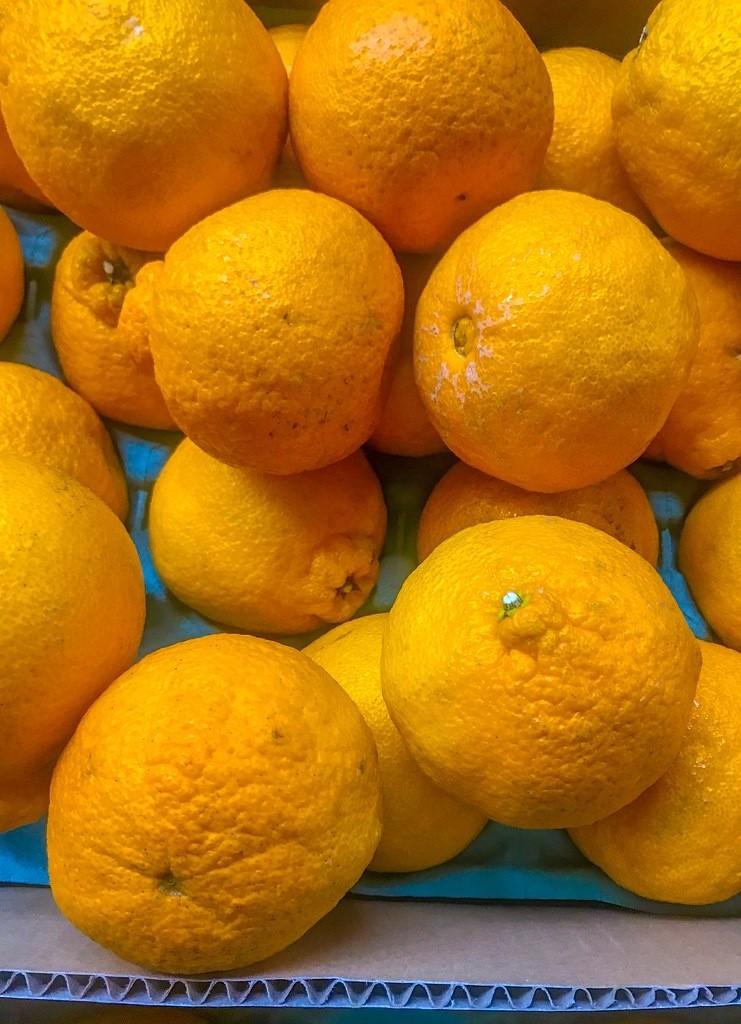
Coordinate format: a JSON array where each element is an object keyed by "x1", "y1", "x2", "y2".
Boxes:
[{"x1": 0, "y1": 0, "x2": 741, "y2": 976}]
